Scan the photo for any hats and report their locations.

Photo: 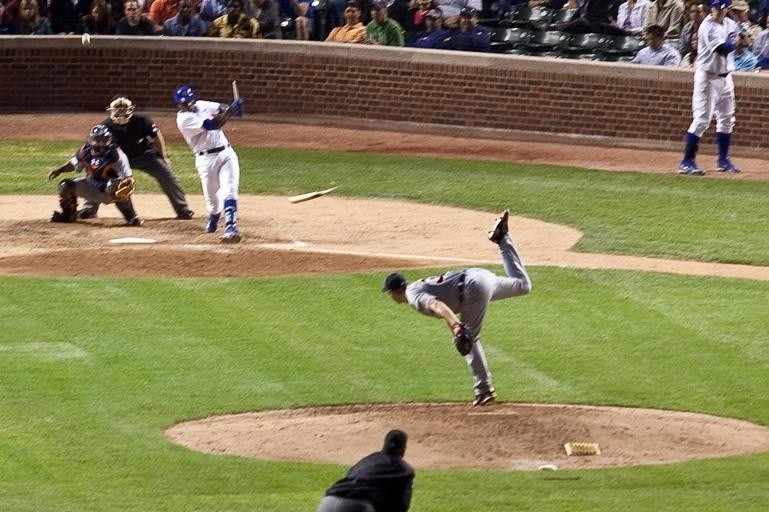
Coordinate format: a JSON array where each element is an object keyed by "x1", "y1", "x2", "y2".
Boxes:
[
  {"x1": 425, "y1": 8, "x2": 441, "y2": 18},
  {"x1": 461, "y1": 7, "x2": 477, "y2": 17},
  {"x1": 382, "y1": 273, "x2": 405, "y2": 292},
  {"x1": 385, "y1": 431, "x2": 407, "y2": 445}
]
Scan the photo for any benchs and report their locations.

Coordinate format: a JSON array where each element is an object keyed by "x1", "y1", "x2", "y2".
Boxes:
[{"x1": 277, "y1": 0, "x2": 646, "y2": 62}]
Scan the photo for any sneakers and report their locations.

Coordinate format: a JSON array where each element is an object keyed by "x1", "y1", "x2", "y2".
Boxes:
[
  {"x1": 178, "y1": 210, "x2": 194, "y2": 218},
  {"x1": 715, "y1": 159, "x2": 740, "y2": 172},
  {"x1": 470, "y1": 391, "x2": 497, "y2": 406},
  {"x1": 489, "y1": 210, "x2": 508, "y2": 244},
  {"x1": 678, "y1": 160, "x2": 704, "y2": 175},
  {"x1": 206, "y1": 213, "x2": 220, "y2": 233},
  {"x1": 219, "y1": 231, "x2": 240, "y2": 243}
]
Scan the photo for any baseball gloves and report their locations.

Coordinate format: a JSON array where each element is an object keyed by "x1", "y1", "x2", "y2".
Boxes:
[
  {"x1": 454, "y1": 322, "x2": 473, "y2": 354},
  {"x1": 744, "y1": 25, "x2": 759, "y2": 47},
  {"x1": 115, "y1": 178, "x2": 134, "y2": 198}
]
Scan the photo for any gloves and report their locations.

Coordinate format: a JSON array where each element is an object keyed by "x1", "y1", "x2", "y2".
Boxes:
[{"x1": 229, "y1": 98, "x2": 243, "y2": 115}]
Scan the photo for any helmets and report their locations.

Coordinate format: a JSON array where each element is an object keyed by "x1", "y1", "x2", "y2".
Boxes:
[
  {"x1": 712, "y1": 0, "x2": 748, "y2": 10},
  {"x1": 90, "y1": 97, "x2": 135, "y2": 158},
  {"x1": 175, "y1": 86, "x2": 197, "y2": 111}
]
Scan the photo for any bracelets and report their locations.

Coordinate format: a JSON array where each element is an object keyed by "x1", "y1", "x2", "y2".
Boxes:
[{"x1": 450, "y1": 321, "x2": 463, "y2": 329}]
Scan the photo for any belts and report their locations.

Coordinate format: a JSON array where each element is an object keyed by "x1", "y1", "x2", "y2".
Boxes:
[
  {"x1": 720, "y1": 73, "x2": 729, "y2": 77},
  {"x1": 199, "y1": 144, "x2": 230, "y2": 155},
  {"x1": 459, "y1": 269, "x2": 466, "y2": 304}
]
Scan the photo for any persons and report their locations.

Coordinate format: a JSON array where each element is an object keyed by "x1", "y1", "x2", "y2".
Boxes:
[
  {"x1": 172, "y1": 83, "x2": 248, "y2": 245},
  {"x1": 0, "y1": 1, "x2": 768, "y2": 79},
  {"x1": 47, "y1": 123, "x2": 145, "y2": 226},
  {"x1": 676, "y1": 1, "x2": 751, "y2": 177},
  {"x1": 315, "y1": 427, "x2": 416, "y2": 512},
  {"x1": 380, "y1": 208, "x2": 534, "y2": 406},
  {"x1": 76, "y1": 93, "x2": 195, "y2": 221}
]
